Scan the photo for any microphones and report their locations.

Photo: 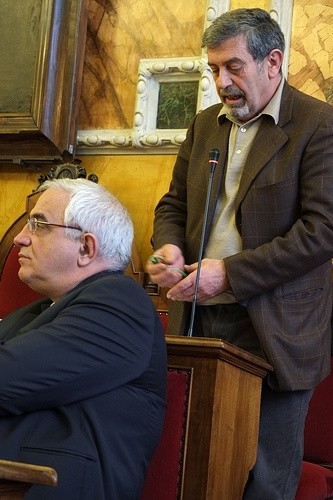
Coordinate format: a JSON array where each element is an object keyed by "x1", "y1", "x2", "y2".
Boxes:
[{"x1": 188, "y1": 149, "x2": 220, "y2": 338}]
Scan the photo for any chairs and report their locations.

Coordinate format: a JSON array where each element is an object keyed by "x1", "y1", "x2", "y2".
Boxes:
[{"x1": 295, "y1": 371, "x2": 333, "y2": 500}]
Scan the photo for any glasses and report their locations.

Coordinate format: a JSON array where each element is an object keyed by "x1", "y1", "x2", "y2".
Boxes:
[{"x1": 27, "y1": 217, "x2": 88, "y2": 234}]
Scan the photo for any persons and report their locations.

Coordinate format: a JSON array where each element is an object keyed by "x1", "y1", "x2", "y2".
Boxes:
[
  {"x1": 145, "y1": 8, "x2": 333, "y2": 500},
  {"x1": 0, "y1": 178, "x2": 168, "y2": 500}
]
